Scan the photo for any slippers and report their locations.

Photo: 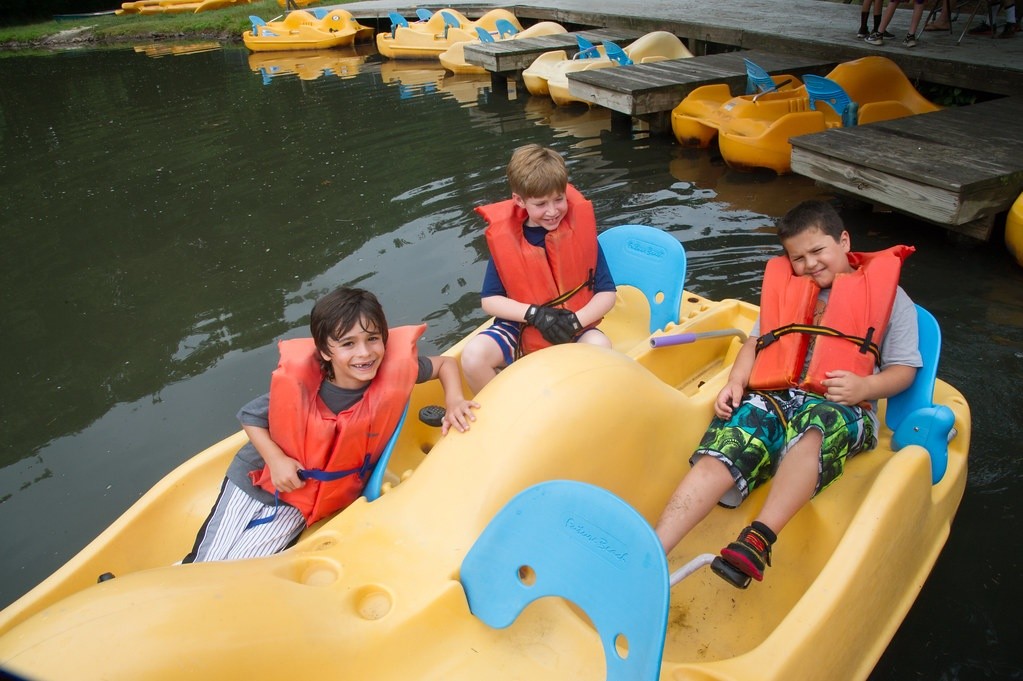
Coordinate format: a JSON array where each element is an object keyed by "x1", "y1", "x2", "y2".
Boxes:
[{"x1": 923, "y1": 20, "x2": 950, "y2": 31}]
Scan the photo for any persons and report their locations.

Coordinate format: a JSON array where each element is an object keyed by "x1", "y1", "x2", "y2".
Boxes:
[
  {"x1": 461, "y1": 144, "x2": 617, "y2": 395},
  {"x1": 174, "y1": 288, "x2": 480, "y2": 564},
  {"x1": 655, "y1": 202, "x2": 923, "y2": 589}
]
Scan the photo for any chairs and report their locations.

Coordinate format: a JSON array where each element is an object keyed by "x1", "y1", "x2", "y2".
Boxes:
[
  {"x1": 475, "y1": 26, "x2": 495, "y2": 43},
  {"x1": 416, "y1": 8, "x2": 433, "y2": 24},
  {"x1": 602, "y1": 38, "x2": 631, "y2": 70},
  {"x1": 804, "y1": 74, "x2": 850, "y2": 122},
  {"x1": 198, "y1": 393, "x2": 410, "y2": 522},
  {"x1": 496, "y1": 19, "x2": 518, "y2": 39},
  {"x1": 314, "y1": 8, "x2": 329, "y2": 20},
  {"x1": 388, "y1": 12, "x2": 409, "y2": 31},
  {"x1": 374, "y1": 480, "x2": 669, "y2": 681},
  {"x1": 442, "y1": 11, "x2": 463, "y2": 33},
  {"x1": 746, "y1": 302, "x2": 956, "y2": 507},
  {"x1": 744, "y1": 58, "x2": 777, "y2": 98},
  {"x1": 577, "y1": 35, "x2": 601, "y2": 60},
  {"x1": 249, "y1": 15, "x2": 266, "y2": 35},
  {"x1": 565, "y1": 222, "x2": 696, "y2": 354}
]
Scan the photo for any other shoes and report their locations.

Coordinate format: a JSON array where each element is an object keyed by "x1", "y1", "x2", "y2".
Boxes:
[
  {"x1": 999, "y1": 19, "x2": 1020, "y2": 38},
  {"x1": 968, "y1": 20, "x2": 997, "y2": 35},
  {"x1": 857, "y1": 28, "x2": 870, "y2": 38},
  {"x1": 878, "y1": 30, "x2": 895, "y2": 40},
  {"x1": 721, "y1": 526, "x2": 775, "y2": 582}
]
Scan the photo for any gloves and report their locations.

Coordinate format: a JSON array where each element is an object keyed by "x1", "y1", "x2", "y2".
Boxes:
[{"x1": 524, "y1": 303, "x2": 584, "y2": 344}]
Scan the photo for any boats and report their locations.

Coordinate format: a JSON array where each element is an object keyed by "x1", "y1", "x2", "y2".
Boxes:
[
  {"x1": 439, "y1": 18, "x2": 569, "y2": 75},
  {"x1": 671, "y1": 55, "x2": 945, "y2": 180},
  {"x1": 250, "y1": 48, "x2": 370, "y2": 85},
  {"x1": 0, "y1": 224, "x2": 973, "y2": 681},
  {"x1": 521, "y1": 30, "x2": 696, "y2": 107},
  {"x1": 377, "y1": 8, "x2": 525, "y2": 60},
  {"x1": 242, "y1": 8, "x2": 374, "y2": 50}
]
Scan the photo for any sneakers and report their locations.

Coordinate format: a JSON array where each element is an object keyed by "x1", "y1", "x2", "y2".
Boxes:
[
  {"x1": 902, "y1": 33, "x2": 917, "y2": 47},
  {"x1": 863, "y1": 33, "x2": 883, "y2": 46}
]
